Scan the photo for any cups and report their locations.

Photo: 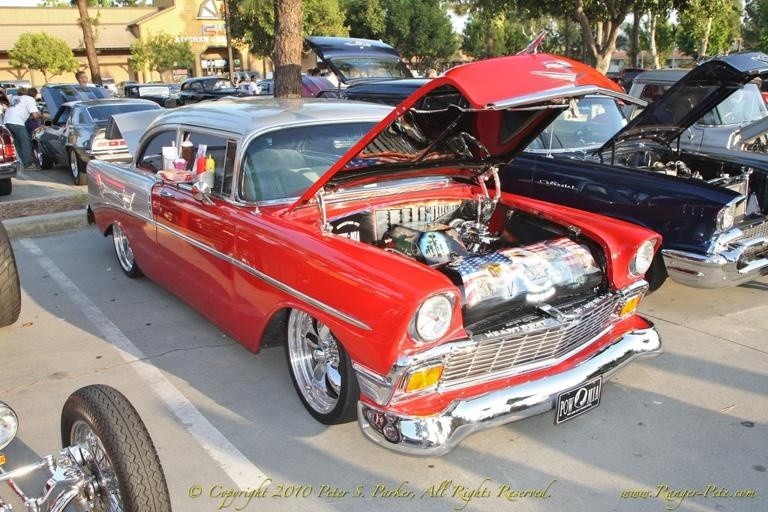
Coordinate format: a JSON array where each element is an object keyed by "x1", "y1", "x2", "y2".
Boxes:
[{"x1": 162, "y1": 146, "x2": 194, "y2": 171}]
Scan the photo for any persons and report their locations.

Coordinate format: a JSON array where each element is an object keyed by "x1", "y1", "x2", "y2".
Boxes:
[
  {"x1": 305, "y1": 64, "x2": 390, "y2": 92},
  {"x1": 74, "y1": 70, "x2": 90, "y2": 86},
  {"x1": 0, "y1": 83, "x2": 51, "y2": 172},
  {"x1": 203, "y1": 74, "x2": 259, "y2": 95},
  {"x1": 608, "y1": 76, "x2": 618, "y2": 83},
  {"x1": 616, "y1": 77, "x2": 628, "y2": 94},
  {"x1": 426, "y1": 68, "x2": 438, "y2": 79}
]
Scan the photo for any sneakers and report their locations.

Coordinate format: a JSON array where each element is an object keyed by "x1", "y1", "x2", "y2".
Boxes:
[{"x1": 23, "y1": 163, "x2": 43, "y2": 173}]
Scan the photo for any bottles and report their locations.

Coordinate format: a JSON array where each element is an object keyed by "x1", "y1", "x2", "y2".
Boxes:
[{"x1": 197, "y1": 153, "x2": 215, "y2": 176}]
